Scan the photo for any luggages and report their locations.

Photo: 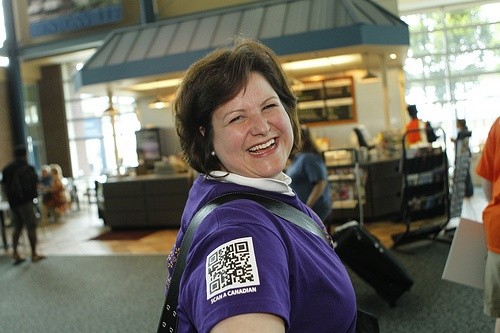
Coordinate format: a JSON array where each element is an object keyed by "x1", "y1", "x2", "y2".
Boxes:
[{"x1": 332, "y1": 221, "x2": 416, "y2": 305}]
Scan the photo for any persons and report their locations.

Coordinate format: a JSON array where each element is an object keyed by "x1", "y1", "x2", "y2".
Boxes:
[
  {"x1": 450, "y1": 118, "x2": 476, "y2": 220},
  {"x1": 477, "y1": 119, "x2": 500, "y2": 331},
  {"x1": 406, "y1": 104, "x2": 438, "y2": 149},
  {"x1": 1, "y1": 145, "x2": 71, "y2": 267},
  {"x1": 166, "y1": 40, "x2": 357, "y2": 333},
  {"x1": 290, "y1": 126, "x2": 332, "y2": 241}
]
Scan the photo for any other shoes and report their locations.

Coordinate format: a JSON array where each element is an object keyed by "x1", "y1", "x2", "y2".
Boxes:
[
  {"x1": 12, "y1": 258, "x2": 25, "y2": 265},
  {"x1": 32, "y1": 255, "x2": 46, "y2": 262}
]
